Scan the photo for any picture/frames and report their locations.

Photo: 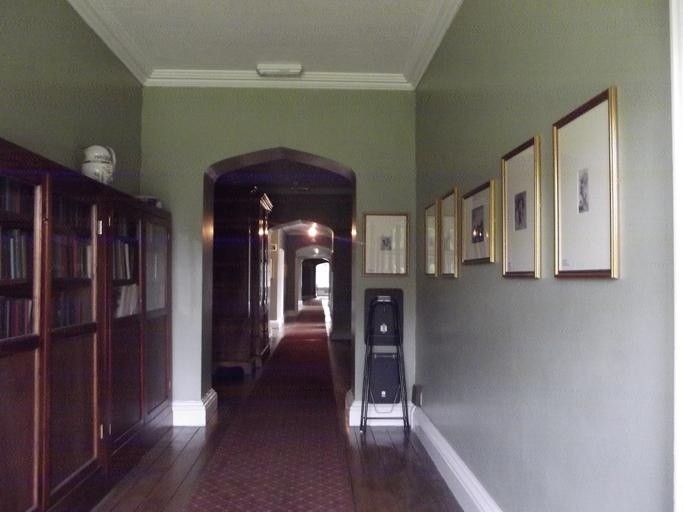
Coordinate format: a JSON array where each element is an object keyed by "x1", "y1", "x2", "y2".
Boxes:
[
  {"x1": 554, "y1": 85, "x2": 617, "y2": 280},
  {"x1": 461, "y1": 181, "x2": 496, "y2": 264},
  {"x1": 423, "y1": 201, "x2": 440, "y2": 278},
  {"x1": 365, "y1": 211, "x2": 408, "y2": 276},
  {"x1": 500, "y1": 136, "x2": 541, "y2": 278},
  {"x1": 441, "y1": 189, "x2": 461, "y2": 279}
]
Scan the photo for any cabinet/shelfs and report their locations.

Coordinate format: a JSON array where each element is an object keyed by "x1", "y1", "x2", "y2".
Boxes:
[
  {"x1": 106, "y1": 184, "x2": 145, "y2": 494},
  {"x1": 45, "y1": 158, "x2": 106, "y2": 511},
  {"x1": 2, "y1": 139, "x2": 45, "y2": 511},
  {"x1": 148, "y1": 201, "x2": 171, "y2": 451}
]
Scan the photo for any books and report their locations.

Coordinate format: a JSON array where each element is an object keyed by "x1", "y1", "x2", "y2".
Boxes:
[{"x1": 0, "y1": 171, "x2": 169, "y2": 338}]
